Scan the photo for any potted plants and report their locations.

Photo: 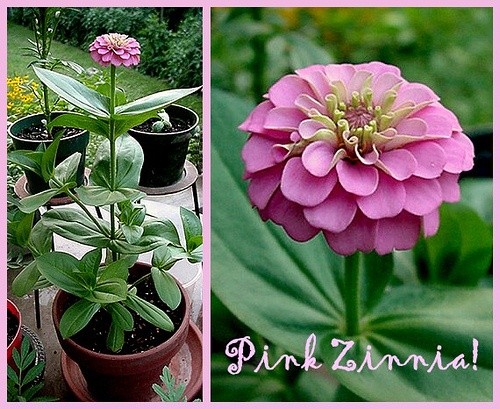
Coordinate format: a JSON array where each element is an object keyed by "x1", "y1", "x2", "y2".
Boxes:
[
  {"x1": 10, "y1": 7, "x2": 88, "y2": 194},
  {"x1": 128, "y1": 104, "x2": 199, "y2": 187}
]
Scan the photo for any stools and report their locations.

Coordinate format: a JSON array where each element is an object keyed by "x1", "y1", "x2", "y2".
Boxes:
[
  {"x1": 128, "y1": 160, "x2": 198, "y2": 225},
  {"x1": 14, "y1": 167, "x2": 104, "y2": 251}
]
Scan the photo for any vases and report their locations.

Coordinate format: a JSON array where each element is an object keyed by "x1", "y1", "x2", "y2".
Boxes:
[
  {"x1": 7, "y1": 301, "x2": 45, "y2": 395},
  {"x1": 52, "y1": 262, "x2": 203, "y2": 402},
  {"x1": 7, "y1": 297, "x2": 22, "y2": 360},
  {"x1": 62, "y1": 322, "x2": 202, "y2": 400}
]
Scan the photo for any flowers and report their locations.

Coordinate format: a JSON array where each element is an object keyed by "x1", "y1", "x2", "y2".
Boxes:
[
  {"x1": 212, "y1": 60, "x2": 493, "y2": 403},
  {"x1": 8, "y1": 34, "x2": 202, "y2": 351}
]
